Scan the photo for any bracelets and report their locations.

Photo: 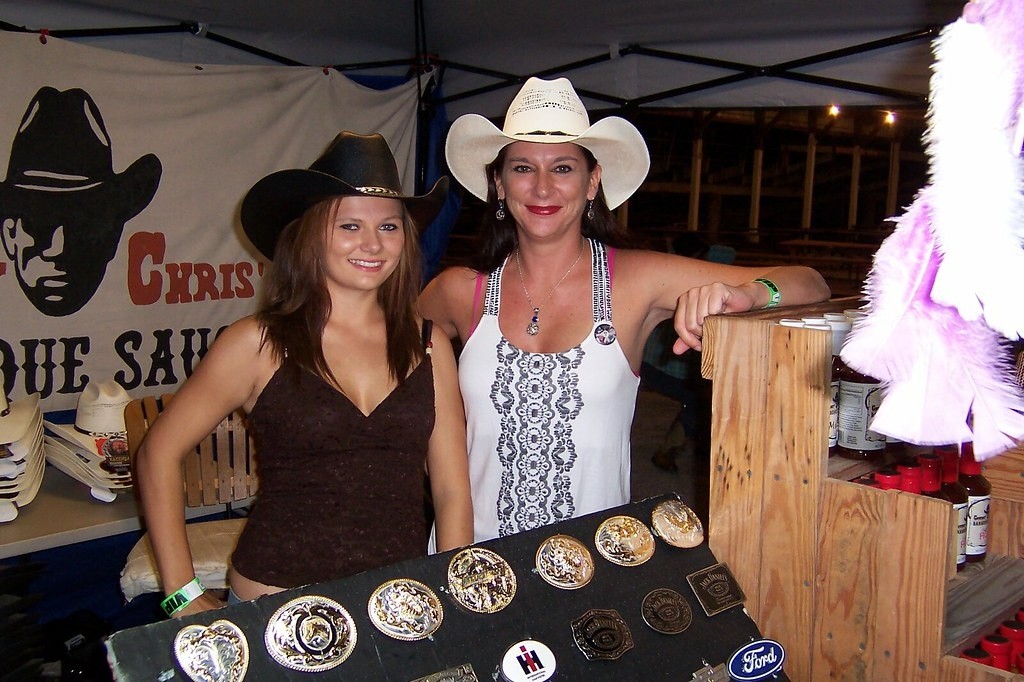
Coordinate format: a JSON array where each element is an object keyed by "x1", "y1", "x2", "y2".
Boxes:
[
  {"x1": 161, "y1": 577, "x2": 207, "y2": 618},
  {"x1": 752, "y1": 278, "x2": 781, "y2": 309}
]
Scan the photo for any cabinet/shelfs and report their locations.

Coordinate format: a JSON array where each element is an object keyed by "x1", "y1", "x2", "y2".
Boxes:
[{"x1": 701, "y1": 301, "x2": 1024, "y2": 682}]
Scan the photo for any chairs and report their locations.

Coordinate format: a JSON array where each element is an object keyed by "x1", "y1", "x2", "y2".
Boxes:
[{"x1": 120, "y1": 390, "x2": 261, "y2": 624}]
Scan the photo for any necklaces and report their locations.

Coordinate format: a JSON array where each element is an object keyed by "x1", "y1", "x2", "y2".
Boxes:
[{"x1": 515, "y1": 236, "x2": 584, "y2": 335}]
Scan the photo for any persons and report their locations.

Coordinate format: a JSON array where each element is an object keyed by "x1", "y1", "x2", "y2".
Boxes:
[
  {"x1": 136, "y1": 132, "x2": 474, "y2": 622},
  {"x1": 414, "y1": 76, "x2": 832, "y2": 556}
]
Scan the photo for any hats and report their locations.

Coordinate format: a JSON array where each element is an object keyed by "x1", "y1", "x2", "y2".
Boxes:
[
  {"x1": 0, "y1": 368, "x2": 45, "y2": 523},
  {"x1": 240, "y1": 130, "x2": 451, "y2": 265},
  {"x1": 44, "y1": 376, "x2": 166, "y2": 502},
  {"x1": 442, "y1": 73, "x2": 652, "y2": 211}
]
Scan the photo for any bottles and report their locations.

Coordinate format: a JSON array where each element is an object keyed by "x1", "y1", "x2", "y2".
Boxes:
[
  {"x1": 778, "y1": 306, "x2": 991, "y2": 569},
  {"x1": 961, "y1": 607, "x2": 1024, "y2": 674}
]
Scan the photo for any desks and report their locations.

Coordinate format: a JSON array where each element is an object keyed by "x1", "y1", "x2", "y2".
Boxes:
[
  {"x1": 780, "y1": 240, "x2": 880, "y2": 283},
  {"x1": 0, "y1": 438, "x2": 259, "y2": 566}
]
